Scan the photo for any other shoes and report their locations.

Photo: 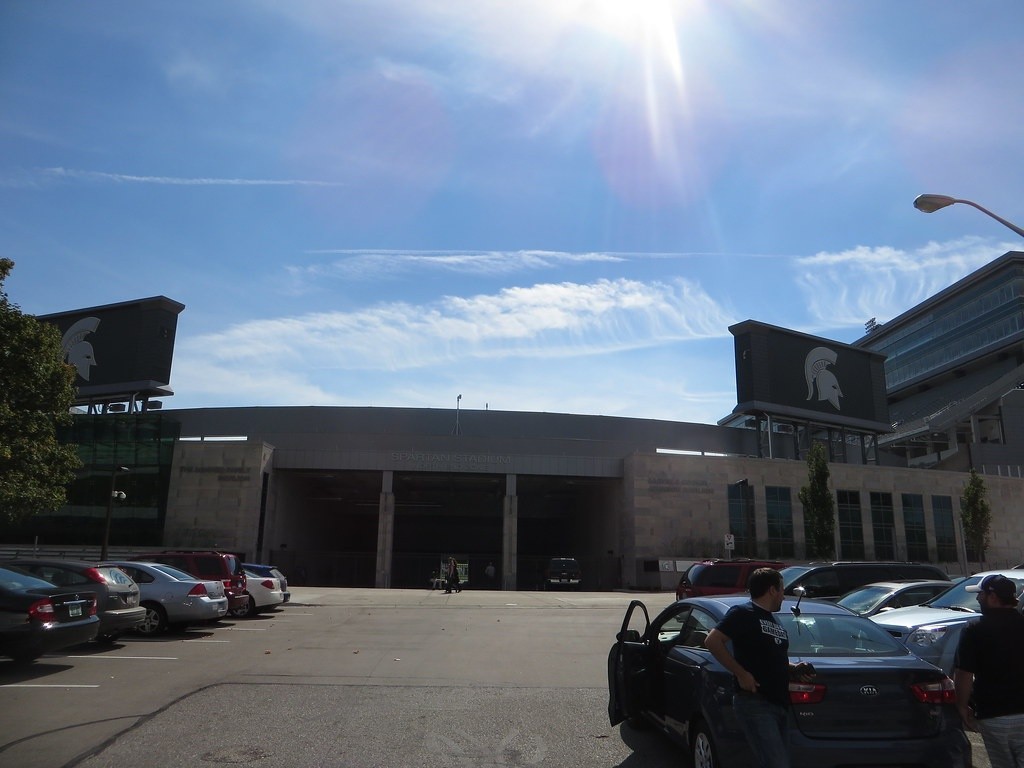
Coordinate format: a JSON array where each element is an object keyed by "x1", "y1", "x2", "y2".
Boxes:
[
  {"x1": 455, "y1": 589, "x2": 462, "y2": 593},
  {"x1": 444, "y1": 590, "x2": 451, "y2": 594}
]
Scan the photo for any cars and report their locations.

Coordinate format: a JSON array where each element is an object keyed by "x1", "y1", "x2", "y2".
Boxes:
[
  {"x1": 131, "y1": 550, "x2": 249, "y2": 624},
  {"x1": 0, "y1": 561, "x2": 100, "y2": 663},
  {"x1": 234, "y1": 563, "x2": 292, "y2": 616},
  {"x1": 671, "y1": 561, "x2": 1024, "y2": 729},
  {"x1": 608, "y1": 592, "x2": 972, "y2": 767},
  {"x1": 112, "y1": 561, "x2": 229, "y2": 633},
  {"x1": 1, "y1": 558, "x2": 147, "y2": 647}
]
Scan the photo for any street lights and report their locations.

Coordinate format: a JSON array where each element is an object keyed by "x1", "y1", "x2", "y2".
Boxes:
[
  {"x1": 98, "y1": 462, "x2": 132, "y2": 557},
  {"x1": 914, "y1": 193, "x2": 1024, "y2": 242}
]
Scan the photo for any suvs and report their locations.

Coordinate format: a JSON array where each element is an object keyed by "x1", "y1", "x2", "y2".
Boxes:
[{"x1": 540, "y1": 557, "x2": 584, "y2": 592}]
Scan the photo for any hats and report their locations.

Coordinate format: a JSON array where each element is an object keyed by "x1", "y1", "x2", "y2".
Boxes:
[{"x1": 964, "y1": 574, "x2": 1019, "y2": 600}]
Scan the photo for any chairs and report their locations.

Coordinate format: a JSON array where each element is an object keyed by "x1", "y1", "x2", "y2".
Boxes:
[
  {"x1": 784, "y1": 621, "x2": 811, "y2": 652},
  {"x1": 822, "y1": 630, "x2": 858, "y2": 651}
]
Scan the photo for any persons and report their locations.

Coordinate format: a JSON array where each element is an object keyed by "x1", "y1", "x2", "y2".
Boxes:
[
  {"x1": 445, "y1": 557, "x2": 462, "y2": 593},
  {"x1": 953, "y1": 574, "x2": 1024, "y2": 768},
  {"x1": 704, "y1": 567, "x2": 817, "y2": 768},
  {"x1": 485, "y1": 562, "x2": 495, "y2": 589}
]
